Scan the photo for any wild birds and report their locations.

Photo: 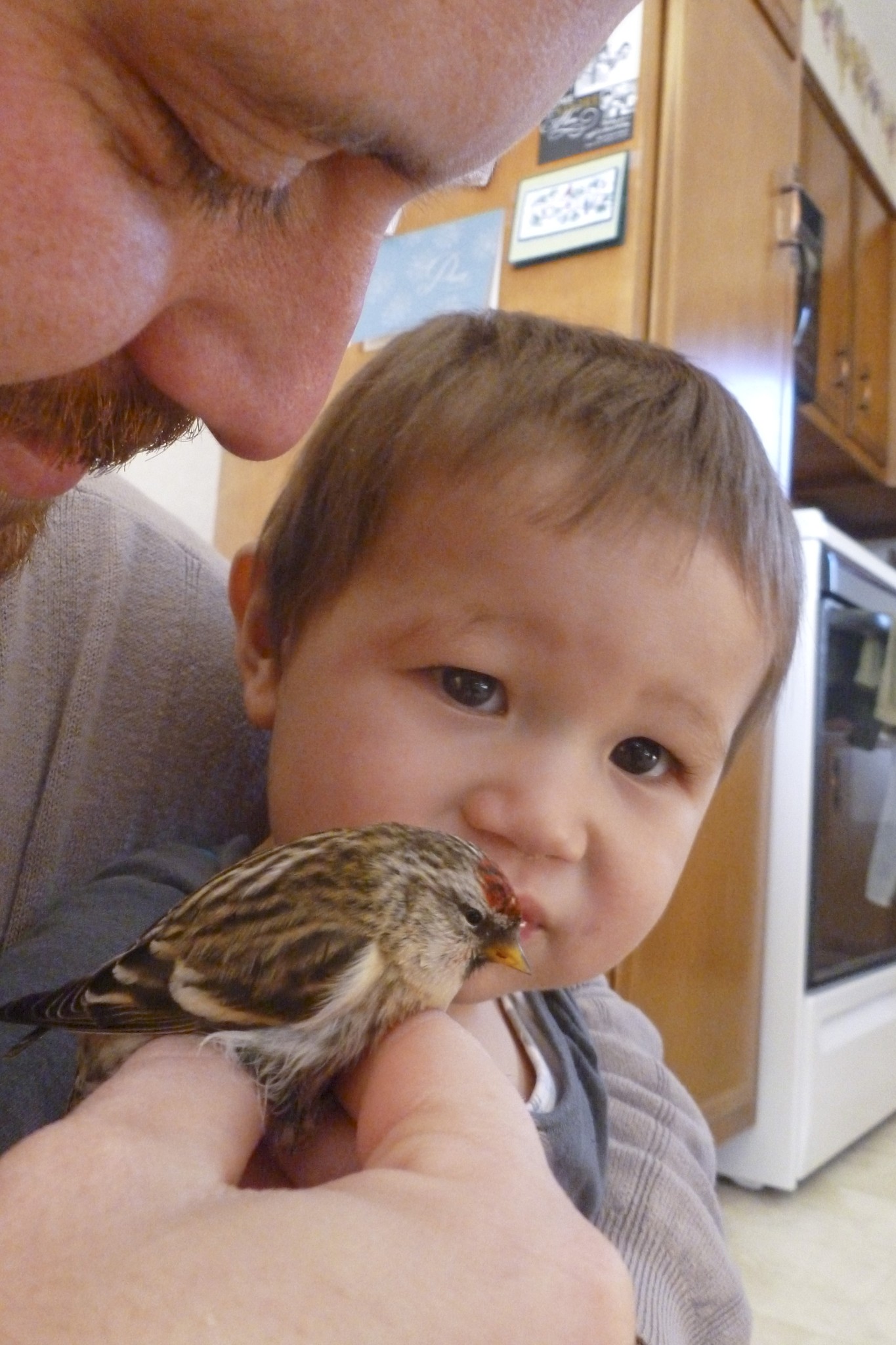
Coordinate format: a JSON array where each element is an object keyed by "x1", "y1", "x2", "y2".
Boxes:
[{"x1": 1, "y1": 823, "x2": 545, "y2": 1189}]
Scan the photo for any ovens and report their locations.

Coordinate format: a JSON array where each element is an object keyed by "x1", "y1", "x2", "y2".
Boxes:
[{"x1": 805, "y1": 544, "x2": 896, "y2": 990}]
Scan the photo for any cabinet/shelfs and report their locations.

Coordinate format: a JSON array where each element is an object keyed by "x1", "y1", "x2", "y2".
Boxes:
[{"x1": 209, "y1": 0, "x2": 896, "y2": 1144}]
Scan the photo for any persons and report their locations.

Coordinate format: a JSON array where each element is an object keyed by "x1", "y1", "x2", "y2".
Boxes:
[
  {"x1": 0, "y1": 0, "x2": 754, "y2": 1345},
  {"x1": 0, "y1": 310, "x2": 806, "y2": 1231}
]
